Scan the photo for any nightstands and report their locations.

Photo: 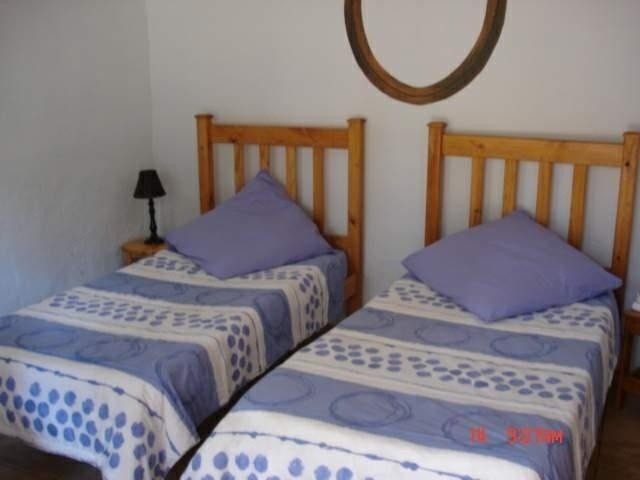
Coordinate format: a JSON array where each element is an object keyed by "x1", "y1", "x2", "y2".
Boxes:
[
  {"x1": 121, "y1": 239, "x2": 169, "y2": 266},
  {"x1": 617, "y1": 307, "x2": 638, "y2": 410}
]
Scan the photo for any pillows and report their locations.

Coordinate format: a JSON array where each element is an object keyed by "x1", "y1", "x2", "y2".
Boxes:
[
  {"x1": 402, "y1": 208, "x2": 623, "y2": 322},
  {"x1": 163, "y1": 169, "x2": 335, "y2": 280}
]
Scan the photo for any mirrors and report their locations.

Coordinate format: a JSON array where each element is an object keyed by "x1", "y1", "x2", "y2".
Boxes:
[{"x1": 339, "y1": 1, "x2": 509, "y2": 107}]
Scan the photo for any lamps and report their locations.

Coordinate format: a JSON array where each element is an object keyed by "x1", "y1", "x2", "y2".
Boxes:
[{"x1": 133, "y1": 167, "x2": 167, "y2": 245}]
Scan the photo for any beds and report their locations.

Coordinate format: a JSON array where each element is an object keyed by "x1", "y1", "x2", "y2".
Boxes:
[
  {"x1": 178, "y1": 122, "x2": 639, "y2": 480},
  {"x1": 0, "y1": 114, "x2": 367, "y2": 479}
]
